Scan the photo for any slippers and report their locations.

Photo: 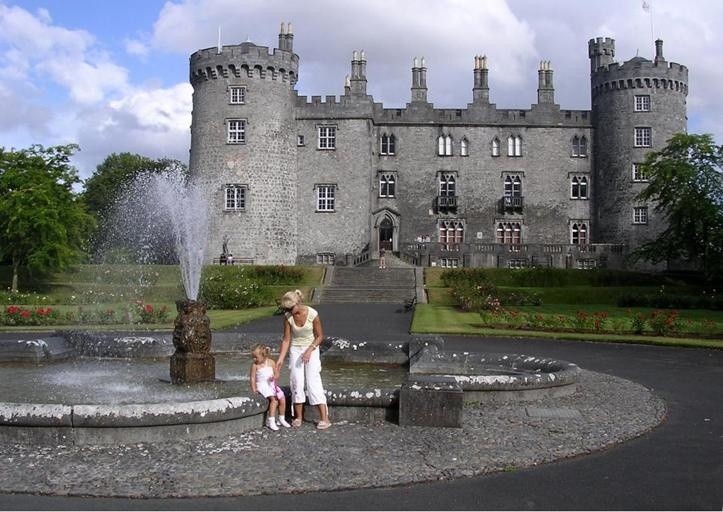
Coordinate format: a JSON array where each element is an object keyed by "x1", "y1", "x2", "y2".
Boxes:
[
  {"x1": 317, "y1": 422, "x2": 332, "y2": 430},
  {"x1": 292, "y1": 419, "x2": 302, "y2": 427}
]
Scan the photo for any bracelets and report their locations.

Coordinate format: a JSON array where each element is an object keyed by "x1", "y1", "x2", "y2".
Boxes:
[{"x1": 309, "y1": 344, "x2": 316, "y2": 350}]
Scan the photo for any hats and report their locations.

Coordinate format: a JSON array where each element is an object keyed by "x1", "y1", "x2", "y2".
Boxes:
[{"x1": 273, "y1": 291, "x2": 299, "y2": 315}]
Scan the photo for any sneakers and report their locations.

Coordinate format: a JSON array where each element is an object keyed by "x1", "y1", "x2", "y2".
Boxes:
[
  {"x1": 266, "y1": 420, "x2": 279, "y2": 431},
  {"x1": 277, "y1": 419, "x2": 291, "y2": 427}
]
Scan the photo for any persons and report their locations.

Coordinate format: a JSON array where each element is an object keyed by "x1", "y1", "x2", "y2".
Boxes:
[
  {"x1": 250, "y1": 344, "x2": 291, "y2": 431},
  {"x1": 222, "y1": 235, "x2": 231, "y2": 254},
  {"x1": 220, "y1": 253, "x2": 227, "y2": 266},
  {"x1": 379, "y1": 248, "x2": 386, "y2": 269},
  {"x1": 275, "y1": 289, "x2": 330, "y2": 430},
  {"x1": 227, "y1": 254, "x2": 234, "y2": 265},
  {"x1": 417, "y1": 234, "x2": 430, "y2": 249}
]
